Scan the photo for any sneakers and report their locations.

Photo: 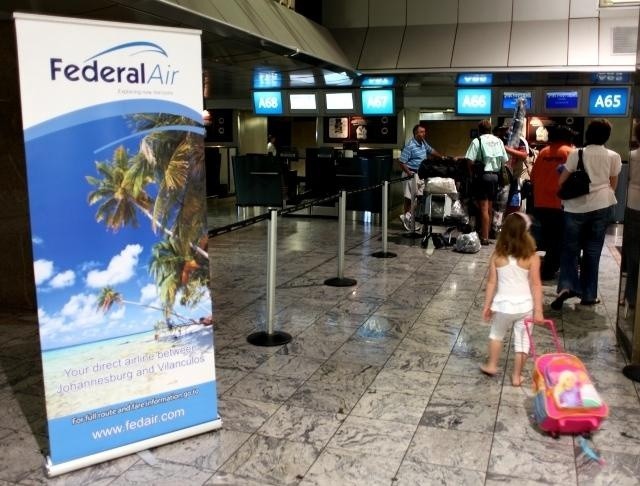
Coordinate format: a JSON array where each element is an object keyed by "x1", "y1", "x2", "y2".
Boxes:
[
  {"x1": 550, "y1": 289, "x2": 570, "y2": 309},
  {"x1": 579, "y1": 297, "x2": 600, "y2": 305},
  {"x1": 399, "y1": 213, "x2": 420, "y2": 231},
  {"x1": 480, "y1": 238, "x2": 489, "y2": 245}
]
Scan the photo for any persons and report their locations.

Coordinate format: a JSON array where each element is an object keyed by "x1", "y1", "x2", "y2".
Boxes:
[
  {"x1": 477, "y1": 211, "x2": 545, "y2": 388},
  {"x1": 619, "y1": 121, "x2": 640, "y2": 309},
  {"x1": 265, "y1": 133, "x2": 277, "y2": 155},
  {"x1": 452, "y1": 117, "x2": 583, "y2": 246},
  {"x1": 550, "y1": 117, "x2": 623, "y2": 311},
  {"x1": 528, "y1": 122, "x2": 576, "y2": 282},
  {"x1": 398, "y1": 124, "x2": 450, "y2": 232},
  {"x1": 551, "y1": 369, "x2": 591, "y2": 410}
]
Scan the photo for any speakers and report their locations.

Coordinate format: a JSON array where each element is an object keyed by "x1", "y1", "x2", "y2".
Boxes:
[
  {"x1": 367, "y1": 116, "x2": 397, "y2": 144},
  {"x1": 209, "y1": 110, "x2": 233, "y2": 142}
]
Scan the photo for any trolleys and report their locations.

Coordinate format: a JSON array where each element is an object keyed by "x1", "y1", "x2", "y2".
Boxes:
[{"x1": 418, "y1": 188, "x2": 466, "y2": 249}]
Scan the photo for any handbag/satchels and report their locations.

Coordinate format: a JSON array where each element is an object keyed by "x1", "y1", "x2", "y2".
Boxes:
[
  {"x1": 499, "y1": 163, "x2": 513, "y2": 186},
  {"x1": 454, "y1": 231, "x2": 481, "y2": 253},
  {"x1": 557, "y1": 148, "x2": 589, "y2": 200},
  {"x1": 415, "y1": 159, "x2": 471, "y2": 224},
  {"x1": 471, "y1": 159, "x2": 485, "y2": 178}
]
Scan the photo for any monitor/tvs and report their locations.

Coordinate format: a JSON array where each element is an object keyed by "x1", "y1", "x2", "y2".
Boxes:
[
  {"x1": 251, "y1": 67, "x2": 398, "y2": 89},
  {"x1": 499, "y1": 90, "x2": 536, "y2": 114},
  {"x1": 323, "y1": 91, "x2": 357, "y2": 114},
  {"x1": 286, "y1": 91, "x2": 318, "y2": 114},
  {"x1": 456, "y1": 73, "x2": 497, "y2": 85},
  {"x1": 584, "y1": 86, "x2": 632, "y2": 117},
  {"x1": 359, "y1": 86, "x2": 405, "y2": 115},
  {"x1": 250, "y1": 91, "x2": 284, "y2": 117},
  {"x1": 542, "y1": 88, "x2": 581, "y2": 113},
  {"x1": 455, "y1": 87, "x2": 493, "y2": 117}
]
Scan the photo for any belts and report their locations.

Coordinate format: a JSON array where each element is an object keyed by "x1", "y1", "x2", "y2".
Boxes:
[{"x1": 484, "y1": 171, "x2": 498, "y2": 174}]
